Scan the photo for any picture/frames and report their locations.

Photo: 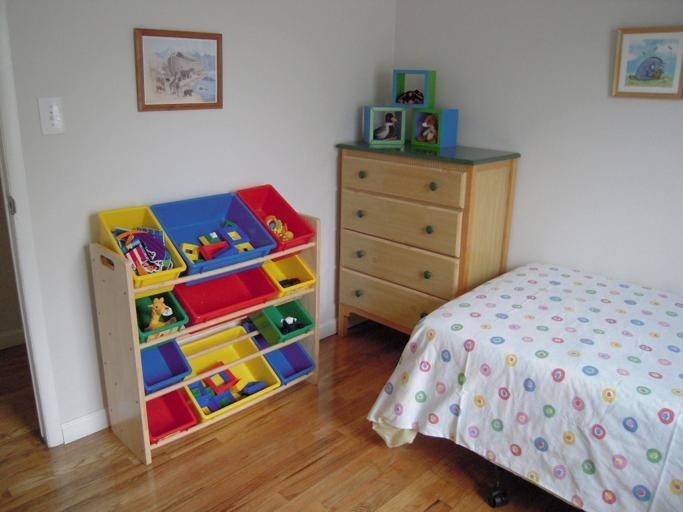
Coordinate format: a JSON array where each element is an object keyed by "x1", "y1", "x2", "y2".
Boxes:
[
  {"x1": 611, "y1": 26, "x2": 683, "y2": 100},
  {"x1": 133, "y1": 28, "x2": 224, "y2": 112}
]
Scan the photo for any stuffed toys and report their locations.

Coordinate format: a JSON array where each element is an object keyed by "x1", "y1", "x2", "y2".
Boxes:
[
  {"x1": 372, "y1": 112, "x2": 399, "y2": 141},
  {"x1": 278, "y1": 315, "x2": 308, "y2": 334},
  {"x1": 144, "y1": 296, "x2": 177, "y2": 331},
  {"x1": 396, "y1": 88, "x2": 423, "y2": 104},
  {"x1": 415, "y1": 114, "x2": 436, "y2": 144}
]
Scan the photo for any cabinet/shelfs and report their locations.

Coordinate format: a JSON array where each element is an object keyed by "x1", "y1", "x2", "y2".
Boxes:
[
  {"x1": 88, "y1": 214, "x2": 321, "y2": 467},
  {"x1": 333, "y1": 141, "x2": 520, "y2": 338}
]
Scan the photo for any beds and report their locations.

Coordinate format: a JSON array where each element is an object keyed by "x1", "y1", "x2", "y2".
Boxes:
[{"x1": 415, "y1": 262, "x2": 681, "y2": 512}]
